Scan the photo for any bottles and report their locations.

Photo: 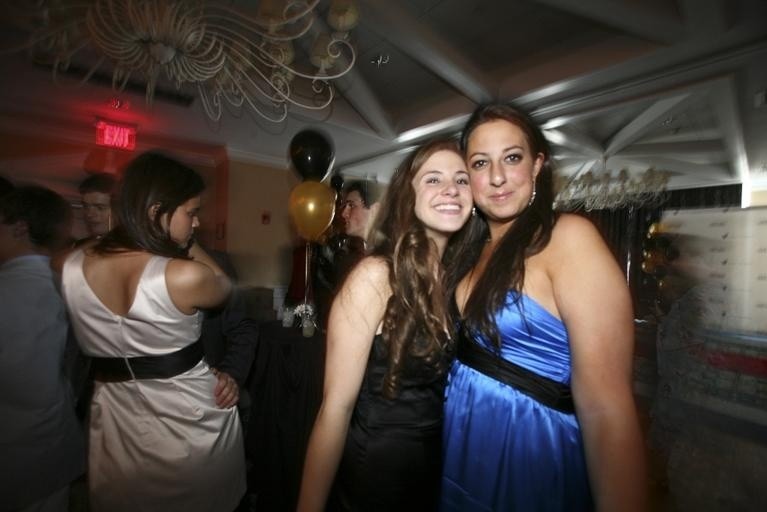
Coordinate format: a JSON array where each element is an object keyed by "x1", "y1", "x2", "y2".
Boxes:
[{"x1": 276, "y1": 306, "x2": 315, "y2": 337}]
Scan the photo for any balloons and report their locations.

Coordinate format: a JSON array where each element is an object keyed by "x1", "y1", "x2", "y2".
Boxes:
[
  {"x1": 285, "y1": 126, "x2": 333, "y2": 184},
  {"x1": 287, "y1": 177, "x2": 340, "y2": 246}
]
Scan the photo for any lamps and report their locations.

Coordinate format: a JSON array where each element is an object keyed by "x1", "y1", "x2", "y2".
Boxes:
[
  {"x1": 550, "y1": 148, "x2": 673, "y2": 215},
  {"x1": 1, "y1": 1, "x2": 362, "y2": 121}
]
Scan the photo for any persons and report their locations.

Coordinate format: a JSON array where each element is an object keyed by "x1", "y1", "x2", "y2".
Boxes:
[
  {"x1": 189, "y1": 232, "x2": 274, "y2": 508},
  {"x1": 637, "y1": 230, "x2": 715, "y2": 435},
  {"x1": 2, "y1": 173, "x2": 119, "y2": 510},
  {"x1": 50, "y1": 150, "x2": 249, "y2": 510},
  {"x1": 438, "y1": 100, "x2": 653, "y2": 511},
  {"x1": 317, "y1": 177, "x2": 390, "y2": 338},
  {"x1": 295, "y1": 134, "x2": 475, "y2": 509}
]
